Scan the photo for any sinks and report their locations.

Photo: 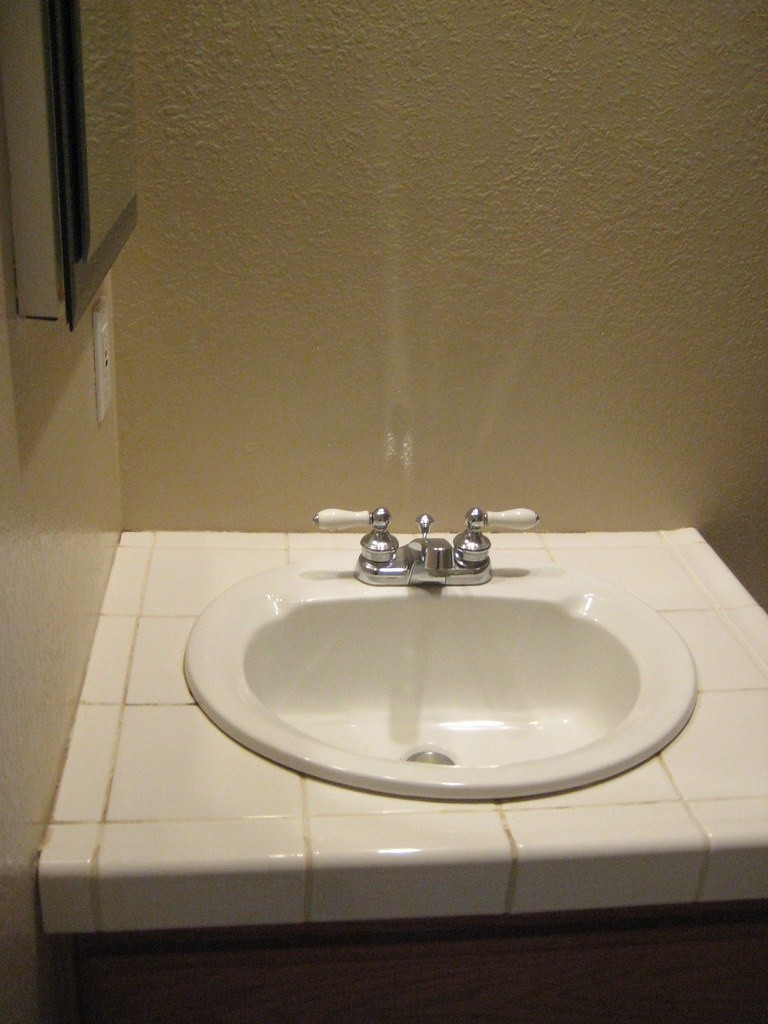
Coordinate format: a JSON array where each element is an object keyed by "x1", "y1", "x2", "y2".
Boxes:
[{"x1": 183, "y1": 556, "x2": 697, "y2": 800}]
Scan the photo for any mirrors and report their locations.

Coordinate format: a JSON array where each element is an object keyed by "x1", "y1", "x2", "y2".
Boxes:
[{"x1": 48, "y1": 0, "x2": 138, "y2": 333}]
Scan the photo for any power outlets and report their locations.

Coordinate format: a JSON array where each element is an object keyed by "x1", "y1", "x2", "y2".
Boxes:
[{"x1": 92, "y1": 293, "x2": 114, "y2": 423}]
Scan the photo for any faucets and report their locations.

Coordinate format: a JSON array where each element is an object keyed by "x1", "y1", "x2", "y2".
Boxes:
[{"x1": 311, "y1": 506, "x2": 540, "y2": 587}]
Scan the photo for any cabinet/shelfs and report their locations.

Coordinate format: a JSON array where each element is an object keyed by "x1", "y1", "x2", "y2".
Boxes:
[{"x1": 59, "y1": 897, "x2": 767, "y2": 1024}]
